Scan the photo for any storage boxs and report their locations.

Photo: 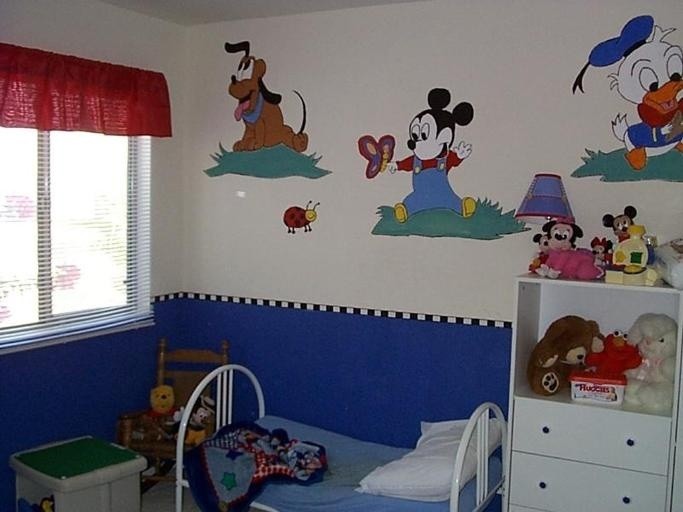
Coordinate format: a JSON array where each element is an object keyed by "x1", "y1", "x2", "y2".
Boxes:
[
  {"x1": 8, "y1": 432, "x2": 148, "y2": 511},
  {"x1": 565, "y1": 371, "x2": 627, "y2": 406}
]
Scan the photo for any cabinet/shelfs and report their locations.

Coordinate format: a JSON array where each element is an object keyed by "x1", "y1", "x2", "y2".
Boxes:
[{"x1": 501, "y1": 269, "x2": 681, "y2": 511}]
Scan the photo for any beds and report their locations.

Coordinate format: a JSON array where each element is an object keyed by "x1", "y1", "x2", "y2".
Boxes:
[{"x1": 174, "y1": 362, "x2": 506, "y2": 512}]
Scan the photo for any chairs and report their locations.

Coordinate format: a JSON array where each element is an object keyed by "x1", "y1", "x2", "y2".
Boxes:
[{"x1": 122, "y1": 332, "x2": 233, "y2": 487}]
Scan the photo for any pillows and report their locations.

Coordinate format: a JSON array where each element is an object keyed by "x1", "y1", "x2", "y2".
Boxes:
[{"x1": 353, "y1": 413, "x2": 501, "y2": 501}]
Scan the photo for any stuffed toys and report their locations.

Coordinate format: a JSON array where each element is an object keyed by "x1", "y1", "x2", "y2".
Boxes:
[
  {"x1": 526, "y1": 313, "x2": 676, "y2": 417},
  {"x1": 528, "y1": 220, "x2": 603, "y2": 280},
  {"x1": 139, "y1": 386, "x2": 216, "y2": 444}
]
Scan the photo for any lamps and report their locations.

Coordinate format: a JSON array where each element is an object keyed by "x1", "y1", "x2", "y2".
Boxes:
[{"x1": 514, "y1": 172, "x2": 575, "y2": 242}]
